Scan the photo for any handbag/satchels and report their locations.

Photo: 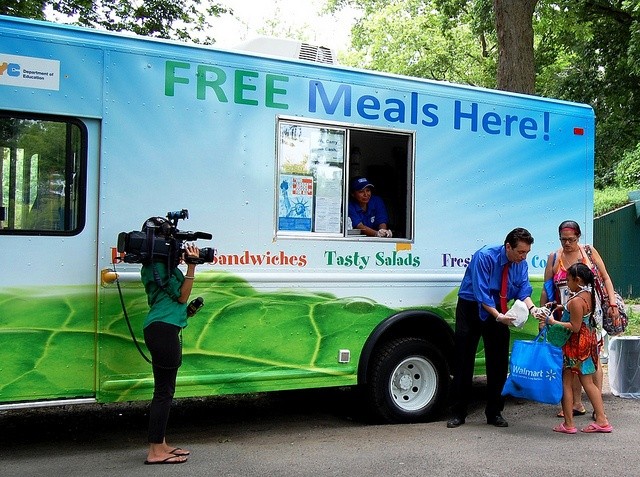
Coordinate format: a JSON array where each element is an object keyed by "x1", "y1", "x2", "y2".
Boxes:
[
  {"x1": 544, "y1": 251, "x2": 558, "y2": 317},
  {"x1": 501, "y1": 324, "x2": 563, "y2": 406},
  {"x1": 583, "y1": 244, "x2": 628, "y2": 336}
]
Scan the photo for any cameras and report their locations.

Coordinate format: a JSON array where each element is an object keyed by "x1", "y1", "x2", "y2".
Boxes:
[{"x1": 116, "y1": 208, "x2": 215, "y2": 264}]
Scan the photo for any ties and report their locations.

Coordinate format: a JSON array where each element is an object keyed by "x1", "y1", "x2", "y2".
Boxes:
[{"x1": 500, "y1": 264, "x2": 509, "y2": 315}]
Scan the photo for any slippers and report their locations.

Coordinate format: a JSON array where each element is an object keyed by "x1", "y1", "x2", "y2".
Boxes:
[
  {"x1": 581, "y1": 421, "x2": 612, "y2": 432},
  {"x1": 144, "y1": 455, "x2": 188, "y2": 465},
  {"x1": 557, "y1": 409, "x2": 586, "y2": 418},
  {"x1": 169, "y1": 448, "x2": 189, "y2": 456},
  {"x1": 553, "y1": 423, "x2": 577, "y2": 433}
]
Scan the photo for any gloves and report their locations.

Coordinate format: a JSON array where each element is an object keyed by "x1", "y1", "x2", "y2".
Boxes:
[
  {"x1": 375, "y1": 229, "x2": 393, "y2": 238},
  {"x1": 496, "y1": 313, "x2": 517, "y2": 326}
]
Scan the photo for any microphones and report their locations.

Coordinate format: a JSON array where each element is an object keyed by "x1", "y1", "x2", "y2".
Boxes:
[{"x1": 187, "y1": 295, "x2": 204, "y2": 317}]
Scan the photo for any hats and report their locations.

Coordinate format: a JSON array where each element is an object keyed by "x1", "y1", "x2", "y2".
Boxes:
[{"x1": 351, "y1": 177, "x2": 374, "y2": 192}]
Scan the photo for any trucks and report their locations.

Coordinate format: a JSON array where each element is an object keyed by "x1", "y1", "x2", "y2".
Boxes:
[{"x1": 0, "y1": 14, "x2": 594, "y2": 422}]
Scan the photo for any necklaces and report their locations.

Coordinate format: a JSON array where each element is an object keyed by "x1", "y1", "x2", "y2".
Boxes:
[{"x1": 563, "y1": 249, "x2": 580, "y2": 266}]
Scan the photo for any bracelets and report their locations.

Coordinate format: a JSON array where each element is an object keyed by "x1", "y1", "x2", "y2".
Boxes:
[
  {"x1": 184, "y1": 272, "x2": 197, "y2": 283},
  {"x1": 494, "y1": 311, "x2": 503, "y2": 323},
  {"x1": 528, "y1": 304, "x2": 536, "y2": 312}
]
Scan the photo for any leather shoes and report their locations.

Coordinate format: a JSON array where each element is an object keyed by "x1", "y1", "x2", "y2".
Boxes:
[
  {"x1": 447, "y1": 417, "x2": 465, "y2": 427},
  {"x1": 487, "y1": 415, "x2": 508, "y2": 427}
]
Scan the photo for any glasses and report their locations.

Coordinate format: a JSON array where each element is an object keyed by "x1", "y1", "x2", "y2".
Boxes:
[{"x1": 560, "y1": 237, "x2": 578, "y2": 242}]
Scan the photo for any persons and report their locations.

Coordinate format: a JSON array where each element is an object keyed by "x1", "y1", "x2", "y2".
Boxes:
[
  {"x1": 541, "y1": 219, "x2": 616, "y2": 421},
  {"x1": 281, "y1": 180, "x2": 309, "y2": 229},
  {"x1": 539, "y1": 263, "x2": 614, "y2": 432},
  {"x1": 348, "y1": 175, "x2": 394, "y2": 237},
  {"x1": 445, "y1": 223, "x2": 542, "y2": 429},
  {"x1": 141, "y1": 213, "x2": 195, "y2": 467}
]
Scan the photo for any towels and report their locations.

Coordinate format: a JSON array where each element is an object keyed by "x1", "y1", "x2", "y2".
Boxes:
[{"x1": 504, "y1": 299, "x2": 530, "y2": 329}]
